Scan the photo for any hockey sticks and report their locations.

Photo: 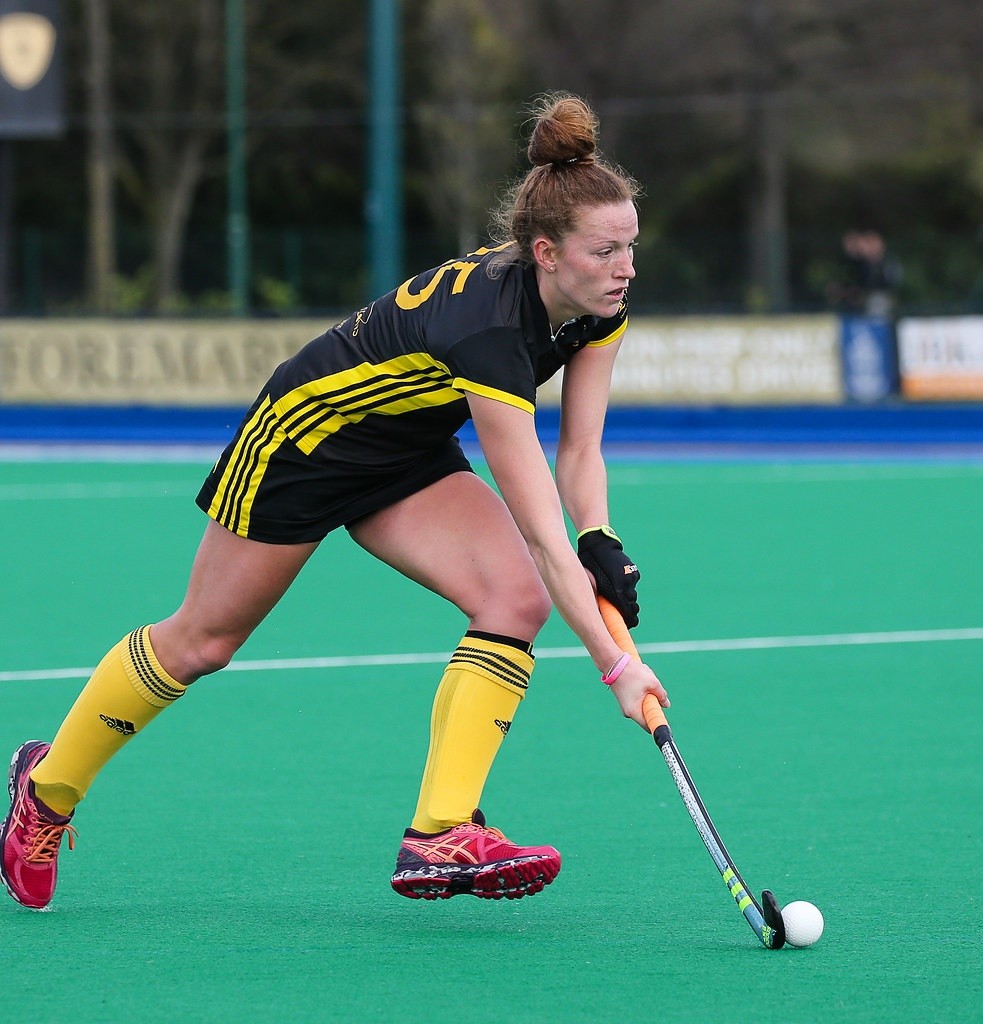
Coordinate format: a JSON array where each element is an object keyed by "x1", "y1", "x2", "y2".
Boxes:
[{"x1": 592, "y1": 595, "x2": 785, "y2": 949}]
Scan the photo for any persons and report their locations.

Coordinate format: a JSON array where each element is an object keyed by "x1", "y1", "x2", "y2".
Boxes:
[{"x1": 3, "y1": 91, "x2": 643, "y2": 916}]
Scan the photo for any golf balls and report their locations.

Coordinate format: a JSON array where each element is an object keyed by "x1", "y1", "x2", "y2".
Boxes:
[{"x1": 780, "y1": 900, "x2": 826, "y2": 948}]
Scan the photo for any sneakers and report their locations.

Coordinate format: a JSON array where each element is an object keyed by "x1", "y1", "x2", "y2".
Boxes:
[
  {"x1": 391, "y1": 807, "x2": 562, "y2": 902},
  {"x1": 0, "y1": 739, "x2": 76, "y2": 910}
]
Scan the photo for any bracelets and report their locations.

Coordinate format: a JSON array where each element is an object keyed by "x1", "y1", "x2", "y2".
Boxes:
[{"x1": 600, "y1": 650, "x2": 631, "y2": 692}]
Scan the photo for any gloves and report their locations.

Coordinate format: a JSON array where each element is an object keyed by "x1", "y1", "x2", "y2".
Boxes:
[{"x1": 574, "y1": 525, "x2": 642, "y2": 630}]
